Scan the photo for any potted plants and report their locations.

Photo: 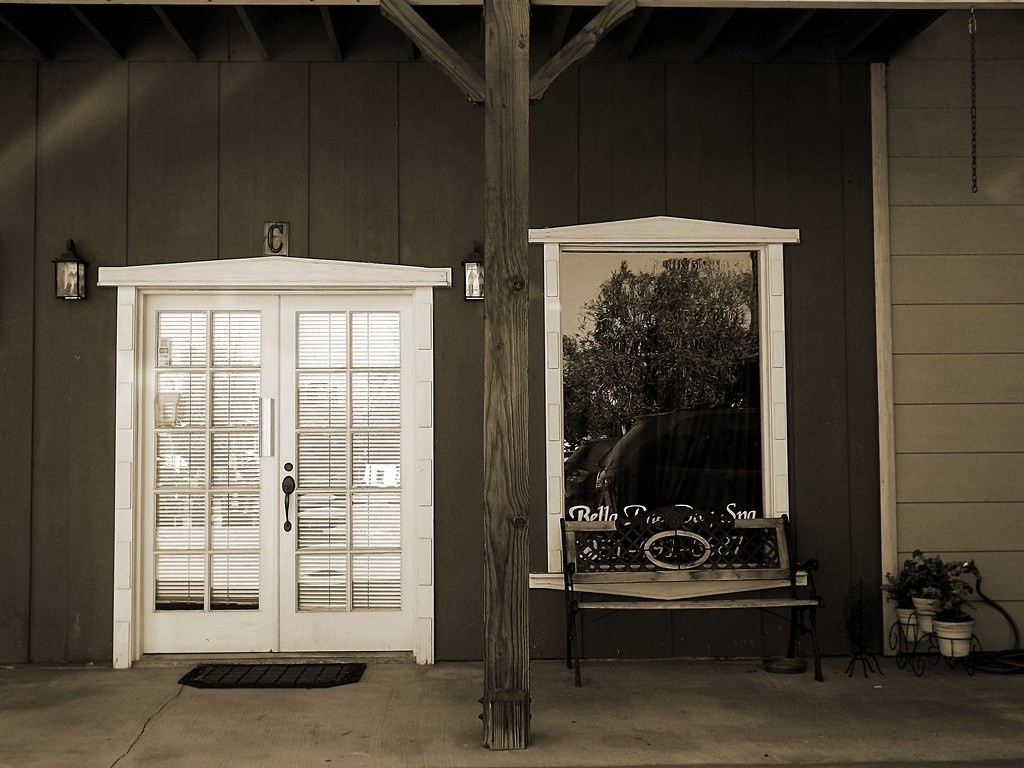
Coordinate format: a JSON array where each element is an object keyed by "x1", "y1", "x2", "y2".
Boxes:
[{"x1": 841, "y1": 549, "x2": 984, "y2": 681}]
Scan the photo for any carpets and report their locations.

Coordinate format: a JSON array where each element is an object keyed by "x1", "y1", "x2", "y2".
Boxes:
[{"x1": 177, "y1": 662, "x2": 367, "y2": 688}]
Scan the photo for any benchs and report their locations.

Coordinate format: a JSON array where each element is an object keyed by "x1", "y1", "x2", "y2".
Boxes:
[{"x1": 561, "y1": 513, "x2": 826, "y2": 688}]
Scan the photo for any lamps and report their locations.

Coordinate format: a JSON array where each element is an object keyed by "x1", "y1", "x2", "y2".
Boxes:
[
  {"x1": 460, "y1": 239, "x2": 486, "y2": 302},
  {"x1": 51, "y1": 238, "x2": 86, "y2": 300}
]
